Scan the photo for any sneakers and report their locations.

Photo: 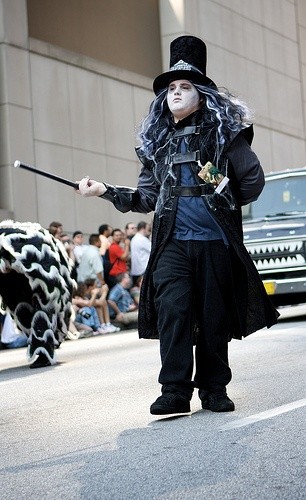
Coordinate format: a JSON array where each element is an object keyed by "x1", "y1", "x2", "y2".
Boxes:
[
  {"x1": 93, "y1": 327, "x2": 108, "y2": 335},
  {"x1": 102, "y1": 322, "x2": 121, "y2": 333}
]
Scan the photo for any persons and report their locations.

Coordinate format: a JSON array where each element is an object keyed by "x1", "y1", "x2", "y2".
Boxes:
[
  {"x1": 73, "y1": 33, "x2": 265, "y2": 415},
  {"x1": 0, "y1": 221, "x2": 154, "y2": 349}
]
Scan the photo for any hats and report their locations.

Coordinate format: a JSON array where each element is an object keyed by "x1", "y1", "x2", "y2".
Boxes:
[{"x1": 152, "y1": 34, "x2": 219, "y2": 96}]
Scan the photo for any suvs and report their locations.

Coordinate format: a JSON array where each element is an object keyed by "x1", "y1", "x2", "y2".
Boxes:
[{"x1": 235, "y1": 165, "x2": 306, "y2": 295}]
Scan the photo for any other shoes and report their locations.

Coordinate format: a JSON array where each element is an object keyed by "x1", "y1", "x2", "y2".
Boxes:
[
  {"x1": 198, "y1": 388, "x2": 236, "y2": 413},
  {"x1": 149, "y1": 393, "x2": 192, "y2": 415}
]
